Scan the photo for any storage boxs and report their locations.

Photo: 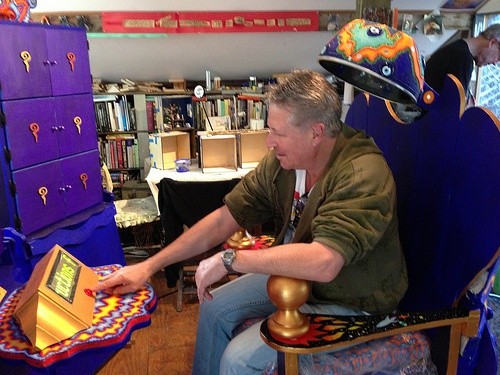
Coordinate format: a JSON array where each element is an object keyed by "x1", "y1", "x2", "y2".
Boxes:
[
  {"x1": 239, "y1": 133, "x2": 268, "y2": 168},
  {"x1": 199, "y1": 135, "x2": 236, "y2": 172},
  {"x1": 149, "y1": 130, "x2": 191, "y2": 170}
]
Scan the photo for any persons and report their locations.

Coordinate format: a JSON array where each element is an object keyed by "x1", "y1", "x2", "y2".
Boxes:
[
  {"x1": 425, "y1": 23, "x2": 500, "y2": 107},
  {"x1": 99, "y1": 70, "x2": 407, "y2": 375}
]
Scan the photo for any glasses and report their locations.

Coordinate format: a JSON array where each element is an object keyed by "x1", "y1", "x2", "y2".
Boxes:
[{"x1": 293, "y1": 192, "x2": 310, "y2": 228}]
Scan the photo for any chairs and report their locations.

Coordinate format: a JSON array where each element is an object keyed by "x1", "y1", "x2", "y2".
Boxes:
[
  {"x1": 156, "y1": 178, "x2": 246, "y2": 312},
  {"x1": 221, "y1": 18, "x2": 500, "y2": 375}
]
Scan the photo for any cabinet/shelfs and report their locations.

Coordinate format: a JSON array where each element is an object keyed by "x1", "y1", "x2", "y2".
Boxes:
[
  {"x1": 93, "y1": 88, "x2": 237, "y2": 171},
  {"x1": 0, "y1": 22, "x2": 105, "y2": 236}
]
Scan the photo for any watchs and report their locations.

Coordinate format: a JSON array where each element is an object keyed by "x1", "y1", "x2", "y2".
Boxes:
[{"x1": 221, "y1": 248, "x2": 238, "y2": 274}]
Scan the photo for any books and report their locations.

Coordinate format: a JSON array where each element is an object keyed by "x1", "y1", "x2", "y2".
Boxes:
[
  {"x1": 95, "y1": 133, "x2": 140, "y2": 168},
  {"x1": 193, "y1": 96, "x2": 267, "y2": 129},
  {"x1": 146, "y1": 97, "x2": 164, "y2": 132},
  {"x1": 93, "y1": 96, "x2": 137, "y2": 132}
]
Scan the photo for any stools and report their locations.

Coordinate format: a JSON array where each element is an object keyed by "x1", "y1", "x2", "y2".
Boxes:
[{"x1": 115, "y1": 196, "x2": 160, "y2": 227}]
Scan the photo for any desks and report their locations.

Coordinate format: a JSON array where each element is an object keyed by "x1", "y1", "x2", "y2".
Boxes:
[{"x1": 147, "y1": 163, "x2": 260, "y2": 208}]
